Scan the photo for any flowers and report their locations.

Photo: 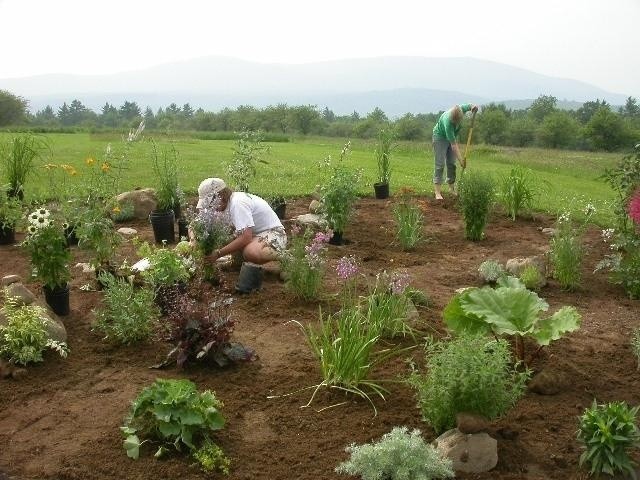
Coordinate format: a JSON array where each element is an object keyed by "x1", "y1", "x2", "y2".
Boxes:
[
  {"x1": 322, "y1": 168, "x2": 363, "y2": 232},
  {"x1": 19, "y1": 207, "x2": 78, "y2": 288},
  {"x1": 373, "y1": 128, "x2": 395, "y2": 183}
]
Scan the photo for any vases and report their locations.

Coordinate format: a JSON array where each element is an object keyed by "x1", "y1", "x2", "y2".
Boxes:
[
  {"x1": 64, "y1": 225, "x2": 79, "y2": 246},
  {"x1": 328, "y1": 230, "x2": 343, "y2": 245},
  {"x1": 273, "y1": 204, "x2": 285, "y2": 220}
]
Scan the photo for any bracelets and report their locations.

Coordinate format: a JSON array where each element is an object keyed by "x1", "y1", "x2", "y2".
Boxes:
[{"x1": 216, "y1": 248, "x2": 222, "y2": 258}]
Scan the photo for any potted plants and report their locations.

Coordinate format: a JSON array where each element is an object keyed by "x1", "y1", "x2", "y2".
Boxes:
[
  {"x1": 373, "y1": 183, "x2": 389, "y2": 199},
  {"x1": 147, "y1": 176, "x2": 178, "y2": 243},
  {"x1": 43, "y1": 282, "x2": 71, "y2": 315},
  {"x1": 0, "y1": 181, "x2": 21, "y2": 244},
  {"x1": 7, "y1": 133, "x2": 46, "y2": 201}
]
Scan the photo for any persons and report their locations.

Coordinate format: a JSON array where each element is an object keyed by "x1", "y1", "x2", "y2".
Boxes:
[
  {"x1": 188, "y1": 178, "x2": 297, "y2": 283},
  {"x1": 431, "y1": 102, "x2": 478, "y2": 200}
]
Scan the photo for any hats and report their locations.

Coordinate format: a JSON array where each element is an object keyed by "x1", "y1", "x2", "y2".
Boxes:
[{"x1": 196, "y1": 177, "x2": 226, "y2": 208}]
